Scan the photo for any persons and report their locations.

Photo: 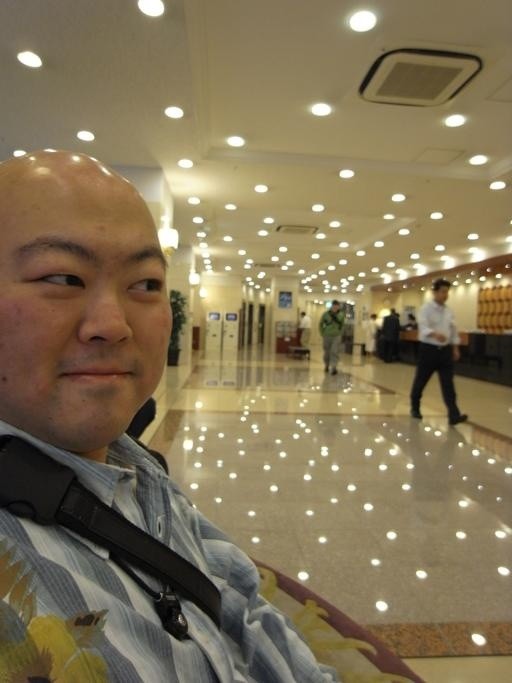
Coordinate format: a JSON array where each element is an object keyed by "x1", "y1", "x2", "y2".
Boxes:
[
  {"x1": 0, "y1": 144, "x2": 337, "y2": 683},
  {"x1": 317, "y1": 299, "x2": 345, "y2": 376},
  {"x1": 363, "y1": 313, "x2": 381, "y2": 359},
  {"x1": 403, "y1": 313, "x2": 416, "y2": 330},
  {"x1": 380, "y1": 307, "x2": 399, "y2": 363},
  {"x1": 408, "y1": 278, "x2": 468, "y2": 427},
  {"x1": 296, "y1": 311, "x2": 313, "y2": 350}
]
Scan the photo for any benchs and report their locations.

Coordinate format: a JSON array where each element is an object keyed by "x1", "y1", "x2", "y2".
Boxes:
[{"x1": 287, "y1": 345, "x2": 311, "y2": 360}]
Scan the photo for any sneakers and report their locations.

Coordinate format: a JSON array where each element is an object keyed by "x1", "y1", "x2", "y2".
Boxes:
[
  {"x1": 325, "y1": 367, "x2": 337, "y2": 375},
  {"x1": 411, "y1": 407, "x2": 421, "y2": 418},
  {"x1": 449, "y1": 415, "x2": 466, "y2": 425}
]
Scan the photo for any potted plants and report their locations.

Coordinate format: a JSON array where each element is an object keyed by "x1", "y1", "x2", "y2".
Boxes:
[{"x1": 167, "y1": 289, "x2": 188, "y2": 365}]
